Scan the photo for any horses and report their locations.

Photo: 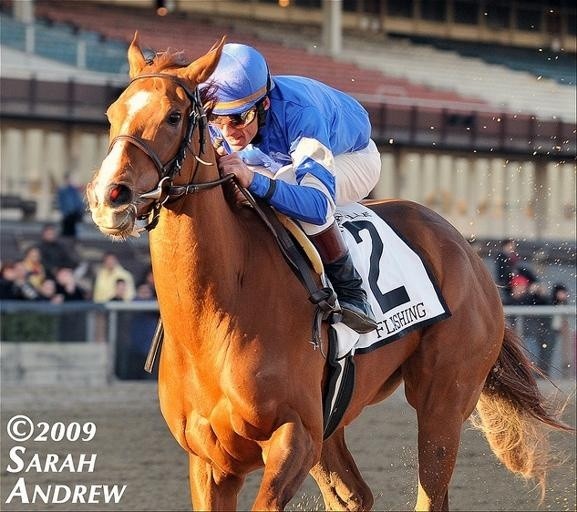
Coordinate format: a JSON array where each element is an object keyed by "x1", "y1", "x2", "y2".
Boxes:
[{"x1": 83, "y1": 31, "x2": 577, "y2": 512}]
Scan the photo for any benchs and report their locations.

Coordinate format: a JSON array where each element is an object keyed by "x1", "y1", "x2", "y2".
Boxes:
[{"x1": 1, "y1": 0, "x2": 577, "y2": 133}]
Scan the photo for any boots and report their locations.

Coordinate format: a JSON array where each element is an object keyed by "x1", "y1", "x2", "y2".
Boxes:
[{"x1": 309, "y1": 220, "x2": 378, "y2": 334}]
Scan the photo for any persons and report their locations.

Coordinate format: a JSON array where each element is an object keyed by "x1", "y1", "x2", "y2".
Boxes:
[
  {"x1": 0, "y1": 167, "x2": 569, "y2": 379},
  {"x1": 196, "y1": 38, "x2": 382, "y2": 334}
]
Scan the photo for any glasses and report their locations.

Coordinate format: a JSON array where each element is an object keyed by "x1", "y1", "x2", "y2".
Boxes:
[{"x1": 209, "y1": 96, "x2": 266, "y2": 129}]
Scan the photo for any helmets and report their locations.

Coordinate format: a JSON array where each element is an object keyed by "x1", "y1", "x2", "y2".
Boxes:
[{"x1": 196, "y1": 43, "x2": 275, "y2": 116}]
